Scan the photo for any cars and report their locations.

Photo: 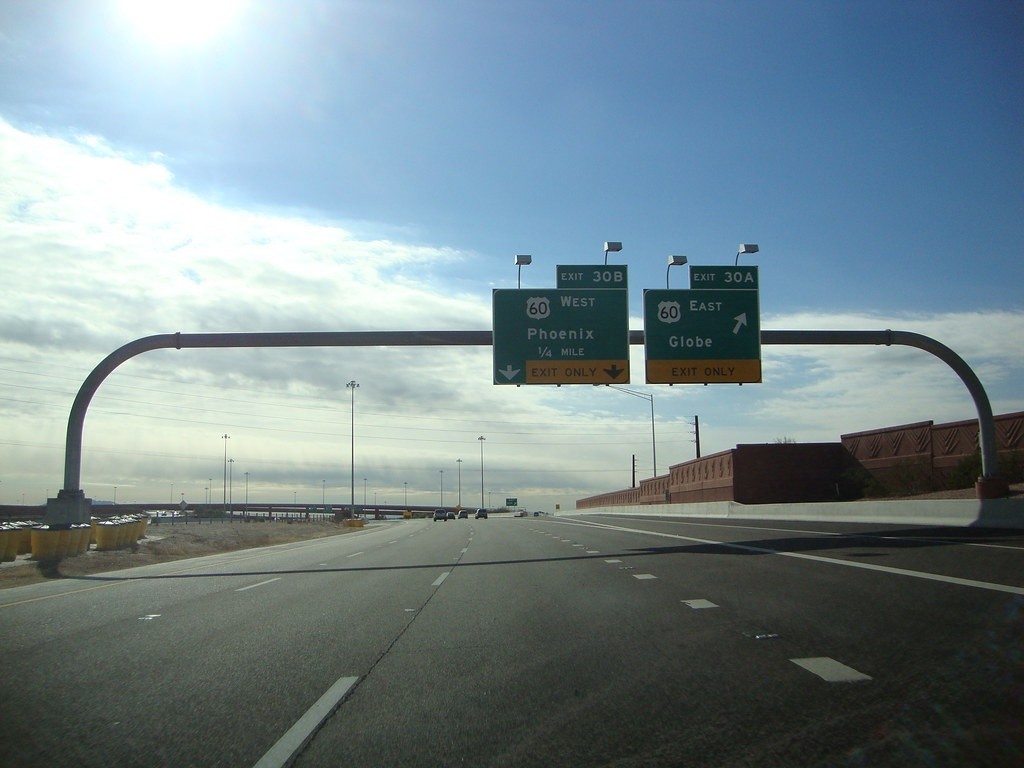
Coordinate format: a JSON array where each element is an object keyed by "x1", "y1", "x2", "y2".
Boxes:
[{"x1": 446, "y1": 512, "x2": 456, "y2": 520}]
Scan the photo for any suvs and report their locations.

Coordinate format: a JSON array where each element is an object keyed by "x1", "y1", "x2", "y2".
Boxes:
[
  {"x1": 458, "y1": 510, "x2": 469, "y2": 520},
  {"x1": 432, "y1": 509, "x2": 447, "y2": 522},
  {"x1": 474, "y1": 509, "x2": 487, "y2": 519}
]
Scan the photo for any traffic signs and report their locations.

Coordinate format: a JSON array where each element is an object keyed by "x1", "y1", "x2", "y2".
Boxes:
[
  {"x1": 506, "y1": 498, "x2": 517, "y2": 506},
  {"x1": 490, "y1": 263, "x2": 633, "y2": 386},
  {"x1": 643, "y1": 263, "x2": 763, "y2": 387}
]
